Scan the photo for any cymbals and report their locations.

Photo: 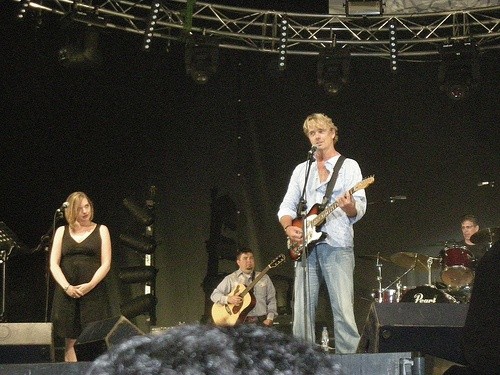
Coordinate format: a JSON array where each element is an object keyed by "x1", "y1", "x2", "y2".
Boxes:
[
  {"x1": 390, "y1": 252, "x2": 440, "y2": 271},
  {"x1": 356, "y1": 254, "x2": 395, "y2": 265},
  {"x1": 470, "y1": 228, "x2": 500, "y2": 248}
]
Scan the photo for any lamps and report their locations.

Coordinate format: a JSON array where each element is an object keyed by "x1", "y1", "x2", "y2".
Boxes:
[
  {"x1": 185, "y1": 38, "x2": 220, "y2": 87},
  {"x1": 316, "y1": 48, "x2": 349, "y2": 98},
  {"x1": 118, "y1": 266, "x2": 156, "y2": 284},
  {"x1": 343, "y1": 0, "x2": 384, "y2": 17},
  {"x1": 440, "y1": 49, "x2": 474, "y2": 99},
  {"x1": 59, "y1": 27, "x2": 97, "y2": 65},
  {"x1": 120, "y1": 228, "x2": 156, "y2": 255},
  {"x1": 119, "y1": 195, "x2": 155, "y2": 227},
  {"x1": 121, "y1": 293, "x2": 157, "y2": 319}
]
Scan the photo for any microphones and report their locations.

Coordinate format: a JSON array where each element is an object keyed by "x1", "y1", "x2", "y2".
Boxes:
[
  {"x1": 308, "y1": 144, "x2": 318, "y2": 155},
  {"x1": 57, "y1": 202, "x2": 70, "y2": 212}
]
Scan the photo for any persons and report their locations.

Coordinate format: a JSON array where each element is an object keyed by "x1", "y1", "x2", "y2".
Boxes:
[
  {"x1": 278, "y1": 113, "x2": 367, "y2": 354},
  {"x1": 457, "y1": 215, "x2": 483, "y2": 258},
  {"x1": 209, "y1": 247, "x2": 279, "y2": 326},
  {"x1": 49, "y1": 191, "x2": 119, "y2": 362}
]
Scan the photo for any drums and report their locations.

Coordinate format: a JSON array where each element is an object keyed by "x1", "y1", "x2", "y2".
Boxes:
[
  {"x1": 399, "y1": 282, "x2": 471, "y2": 304},
  {"x1": 372, "y1": 288, "x2": 398, "y2": 304},
  {"x1": 440, "y1": 244, "x2": 476, "y2": 287}
]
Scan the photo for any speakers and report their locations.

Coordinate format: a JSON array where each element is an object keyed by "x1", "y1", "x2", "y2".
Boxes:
[
  {"x1": 354, "y1": 303, "x2": 470, "y2": 364},
  {"x1": 0, "y1": 323, "x2": 55, "y2": 363},
  {"x1": 73, "y1": 315, "x2": 146, "y2": 362}
]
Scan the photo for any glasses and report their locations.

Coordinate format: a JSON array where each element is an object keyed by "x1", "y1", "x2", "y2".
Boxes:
[{"x1": 461, "y1": 225, "x2": 475, "y2": 231}]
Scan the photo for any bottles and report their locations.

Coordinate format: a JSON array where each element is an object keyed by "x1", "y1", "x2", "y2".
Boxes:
[{"x1": 322, "y1": 327, "x2": 329, "y2": 350}]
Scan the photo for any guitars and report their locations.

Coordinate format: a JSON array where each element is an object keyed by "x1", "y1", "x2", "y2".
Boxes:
[
  {"x1": 287, "y1": 174, "x2": 374, "y2": 262},
  {"x1": 210, "y1": 253, "x2": 286, "y2": 328}
]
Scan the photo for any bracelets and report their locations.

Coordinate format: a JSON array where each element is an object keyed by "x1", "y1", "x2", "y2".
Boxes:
[
  {"x1": 285, "y1": 225, "x2": 290, "y2": 229},
  {"x1": 64, "y1": 283, "x2": 70, "y2": 292}
]
